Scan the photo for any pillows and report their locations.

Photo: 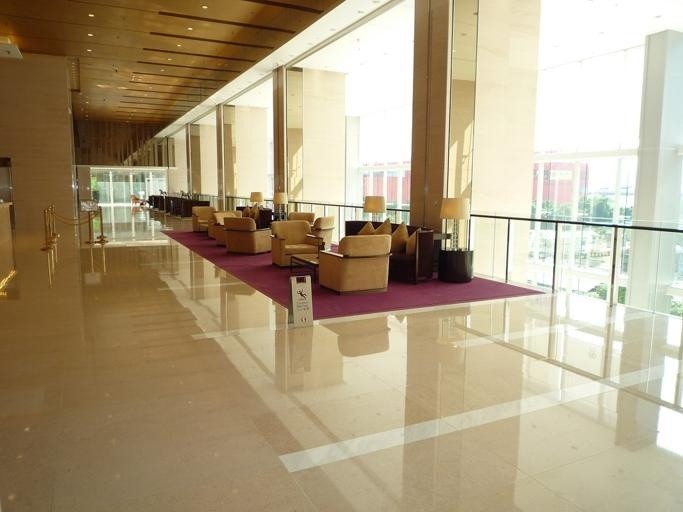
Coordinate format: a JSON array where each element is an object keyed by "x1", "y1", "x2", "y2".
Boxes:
[
  {"x1": 242, "y1": 203, "x2": 263, "y2": 226},
  {"x1": 370, "y1": 217, "x2": 391, "y2": 235}
]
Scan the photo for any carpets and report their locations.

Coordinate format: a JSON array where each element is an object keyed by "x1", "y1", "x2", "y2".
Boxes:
[{"x1": 159, "y1": 231, "x2": 546, "y2": 321}]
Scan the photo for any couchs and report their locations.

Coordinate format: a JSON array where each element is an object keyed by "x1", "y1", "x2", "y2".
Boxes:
[{"x1": 344, "y1": 221, "x2": 433, "y2": 285}]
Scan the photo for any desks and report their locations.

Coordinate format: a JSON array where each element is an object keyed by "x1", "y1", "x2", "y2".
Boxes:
[{"x1": 438, "y1": 247, "x2": 473, "y2": 283}]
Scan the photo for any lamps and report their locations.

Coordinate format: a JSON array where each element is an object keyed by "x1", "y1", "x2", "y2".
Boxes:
[
  {"x1": 439, "y1": 197, "x2": 470, "y2": 251},
  {"x1": 363, "y1": 195, "x2": 386, "y2": 221},
  {"x1": 249, "y1": 192, "x2": 264, "y2": 208},
  {"x1": 272, "y1": 192, "x2": 288, "y2": 221}
]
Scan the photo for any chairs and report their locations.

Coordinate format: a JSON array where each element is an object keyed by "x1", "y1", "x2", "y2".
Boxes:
[{"x1": 191, "y1": 206, "x2": 392, "y2": 296}]
